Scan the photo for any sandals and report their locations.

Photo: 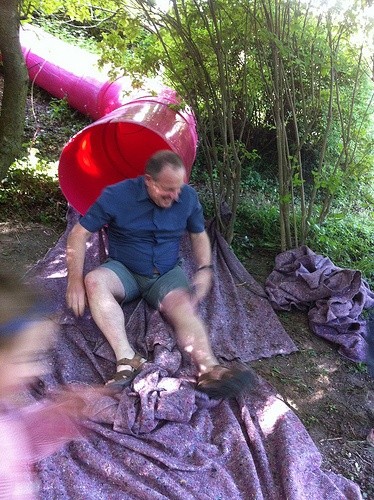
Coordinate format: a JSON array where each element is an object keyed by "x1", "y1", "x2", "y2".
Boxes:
[
  {"x1": 105, "y1": 352, "x2": 146, "y2": 388},
  {"x1": 196, "y1": 367, "x2": 252, "y2": 398}
]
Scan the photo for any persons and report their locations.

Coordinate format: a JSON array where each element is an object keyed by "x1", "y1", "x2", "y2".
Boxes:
[
  {"x1": 1, "y1": 273, "x2": 123, "y2": 498},
  {"x1": 62, "y1": 150, "x2": 246, "y2": 409}
]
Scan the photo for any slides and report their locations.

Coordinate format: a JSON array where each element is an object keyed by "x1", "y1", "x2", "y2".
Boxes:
[{"x1": 19, "y1": 24, "x2": 197, "y2": 228}]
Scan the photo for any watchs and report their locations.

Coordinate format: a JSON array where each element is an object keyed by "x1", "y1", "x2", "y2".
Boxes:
[{"x1": 194, "y1": 262, "x2": 217, "y2": 274}]
